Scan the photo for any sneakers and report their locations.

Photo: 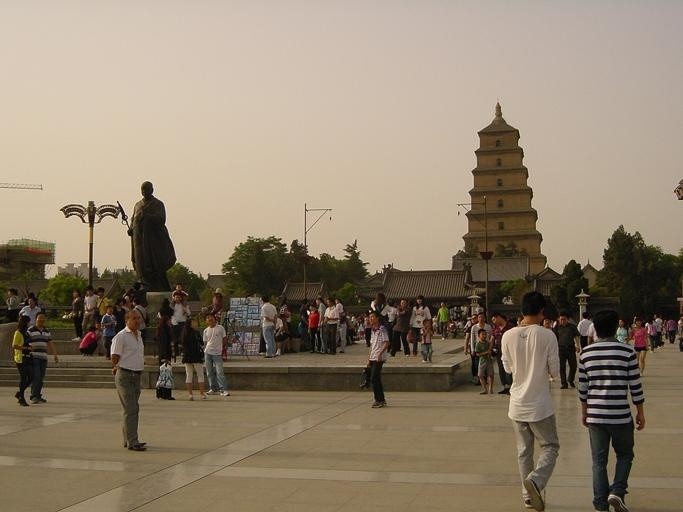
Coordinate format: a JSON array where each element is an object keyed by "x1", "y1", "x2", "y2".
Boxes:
[
  {"x1": 219, "y1": 390, "x2": 230, "y2": 396},
  {"x1": 523, "y1": 478, "x2": 545, "y2": 512},
  {"x1": 259, "y1": 348, "x2": 297, "y2": 357},
  {"x1": 648, "y1": 338, "x2": 676, "y2": 353},
  {"x1": 480, "y1": 389, "x2": 493, "y2": 394},
  {"x1": 345, "y1": 336, "x2": 370, "y2": 348},
  {"x1": 560, "y1": 381, "x2": 575, "y2": 389},
  {"x1": 388, "y1": 351, "x2": 395, "y2": 357},
  {"x1": 206, "y1": 389, "x2": 218, "y2": 395},
  {"x1": 372, "y1": 399, "x2": 387, "y2": 408},
  {"x1": 423, "y1": 358, "x2": 431, "y2": 364},
  {"x1": 15, "y1": 392, "x2": 28, "y2": 405},
  {"x1": 310, "y1": 349, "x2": 345, "y2": 355},
  {"x1": 498, "y1": 387, "x2": 510, "y2": 396},
  {"x1": 608, "y1": 493, "x2": 630, "y2": 511},
  {"x1": 72, "y1": 337, "x2": 82, "y2": 341},
  {"x1": 592, "y1": 498, "x2": 610, "y2": 511},
  {"x1": 155, "y1": 393, "x2": 176, "y2": 400},
  {"x1": 405, "y1": 352, "x2": 417, "y2": 358},
  {"x1": 30, "y1": 395, "x2": 47, "y2": 404}
]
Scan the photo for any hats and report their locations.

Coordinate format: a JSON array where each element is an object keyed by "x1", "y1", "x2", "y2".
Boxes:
[{"x1": 214, "y1": 288, "x2": 225, "y2": 296}]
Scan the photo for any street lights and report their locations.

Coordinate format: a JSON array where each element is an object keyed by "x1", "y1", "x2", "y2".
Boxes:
[
  {"x1": 59, "y1": 198, "x2": 123, "y2": 291},
  {"x1": 478, "y1": 250, "x2": 495, "y2": 320},
  {"x1": 576, "y1": 288, "x2": 590, "y2": 320}
]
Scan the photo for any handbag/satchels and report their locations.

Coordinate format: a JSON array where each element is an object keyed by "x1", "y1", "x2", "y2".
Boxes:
[
  {"x1": 360, "y1": 366, "x2": 371, "y2": 388},
  {"x1": 155, "y1": 364, "x2": 174, "y2": 389},
  {"x1": 93, "y1": 308, "x2": 99, "y2": 318}
]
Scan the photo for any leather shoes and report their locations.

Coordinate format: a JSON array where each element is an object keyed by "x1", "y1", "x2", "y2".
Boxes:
[
  {"x1": 187, "y1": 394, "x2": 193, "y2": 401},
  {"x1": 200, "y1": 393, "x2": 208, "y2": 400},
  {"x1": 124, "y1": 442, "x2": 147, "y2": 452}
]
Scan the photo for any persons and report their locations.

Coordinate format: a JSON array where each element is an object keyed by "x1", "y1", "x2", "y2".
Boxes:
[
  {"x1": 576, "y1": 310, "x2": 645, "y2": 512},
  {"x1": 109, "y1": 307, "x2": 146, "y2": 452},
  {"x1": 499, "y1": 291, "x2": 564, "y2": 511},
  {"x1": 126, "y1": 182, "x2": 177, "y2": 293}
]
